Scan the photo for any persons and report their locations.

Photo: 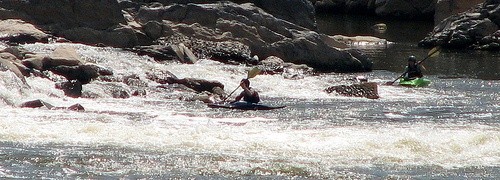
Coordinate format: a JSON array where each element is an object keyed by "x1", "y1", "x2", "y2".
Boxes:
[
  {"x1": 225, "y1": 78, "x2": 260, "y2": 105},
  {"x1": 399, "y1": 55, "x2": 427, "y2": 82}
]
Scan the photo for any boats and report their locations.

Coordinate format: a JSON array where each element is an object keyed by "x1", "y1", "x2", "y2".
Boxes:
[
  {"x1": 399, "y1": 76, "x2": 432, "y2": 88},
  {"x1": 207, "y1": 99, "x2": 287, "y2": 111}
]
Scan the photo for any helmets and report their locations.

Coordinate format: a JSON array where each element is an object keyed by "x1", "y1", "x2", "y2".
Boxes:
[{"x1": 408, "y1": 56, "x2": 417, "y2": 62}]
